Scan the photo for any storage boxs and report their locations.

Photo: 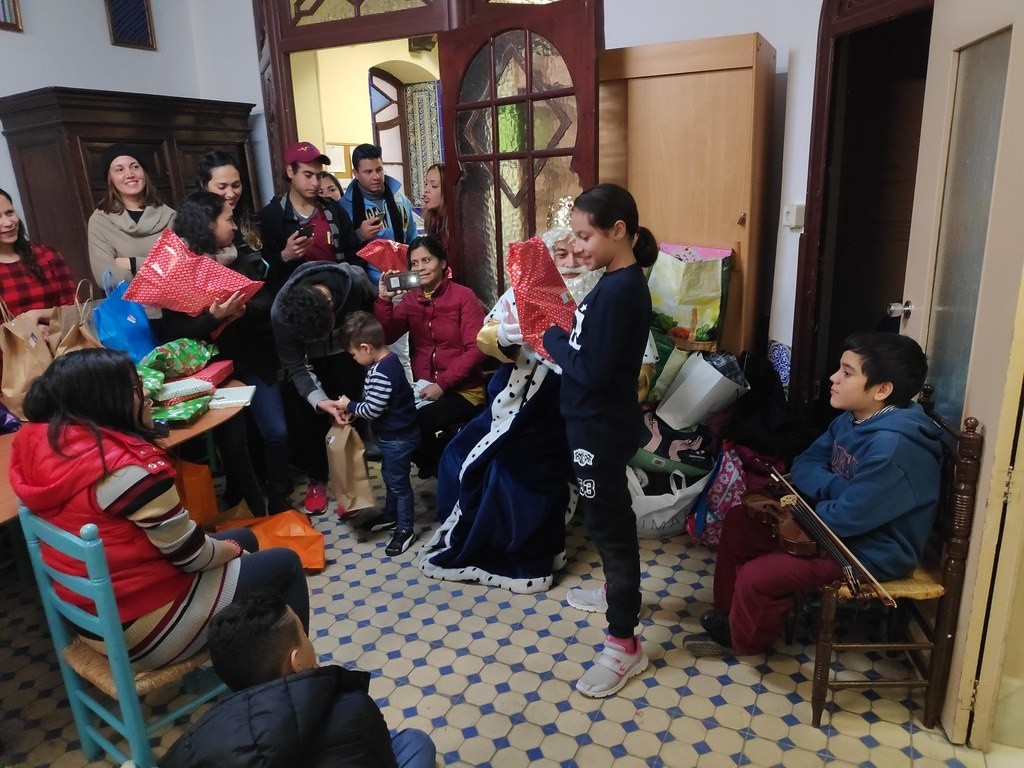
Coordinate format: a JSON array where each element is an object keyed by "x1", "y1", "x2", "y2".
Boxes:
[{"x1": 149, "y1": 359, "x2": 234, "y2": 428}]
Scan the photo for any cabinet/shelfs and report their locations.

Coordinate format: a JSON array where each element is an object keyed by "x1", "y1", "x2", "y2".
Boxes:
[
  {"x1": 1, "y1": 86, "x2": 265, "y2": 301},
  {"x1": 598, "y1": 32, "x2": 778, "y2": 360}
]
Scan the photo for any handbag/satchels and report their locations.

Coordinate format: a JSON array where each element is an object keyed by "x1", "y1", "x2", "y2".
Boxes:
[
  {"x1": 1, "y1": 273, "x2": 218, "y2": 424},
  {"x1": 509, "y1": 236, "x2": 577, "y2": 365},
  {"x1": 624, "y1": 243, "x2": 749, "y2": 549},
  {"x1": 215, "y1": 511, "x2": 326, "y2": 571},
  {"x1": 172, "y1": 461, "x2": 218, "y2": 535},
  {"x1": 120, "y1": 229, "x2": 264, "y2": 316}
]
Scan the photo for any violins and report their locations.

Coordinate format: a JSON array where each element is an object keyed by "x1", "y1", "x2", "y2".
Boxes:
[{"x1": 740, "y1": 462, "x2": 899, "y2": 612}]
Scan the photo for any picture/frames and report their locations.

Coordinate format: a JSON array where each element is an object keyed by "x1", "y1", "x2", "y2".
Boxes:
[
  {"x1": 104, "y1": 0, "x2": 157, "y2": 51},
  {"x1": 0, "y1": 0, "x2": 23, "y2": 32}
]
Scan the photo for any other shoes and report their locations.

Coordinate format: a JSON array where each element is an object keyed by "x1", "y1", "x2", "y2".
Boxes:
[
  {"x1": 418, "y1": 469, "x2": 433, "y2": 479},
  {"x1": 221, "y1": 486, "x2": 243, "y2": 509},
  {"x1": 365, "y1": 443, "x2": 383, "y2": 461},
  {"x1": 699, "y1": 610, "x2": 732, "y2": 648},
  {"x1": 683, "y1": 634, "x2": 728, "y2": 656}
]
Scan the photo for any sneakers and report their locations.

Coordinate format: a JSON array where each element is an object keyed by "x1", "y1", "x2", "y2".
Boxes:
[
  {"x1": 360, "y1": 512, "x2": 397, "y2": 533},
  {"x1": 336, "y1": 501, "x2": 360, "y2": 520},
  {"x1": 385, "y1": 530, "x2": 416, "y2": 556},
  {"x1": 576, "y1": 638, "x2": 648, "y2": 698},
  {"x1": 565, "y1": 583, "x2": 641, "y2": 618},
  {"x1": 304, "y1": 482, "x2": 329, "y2": 514}
]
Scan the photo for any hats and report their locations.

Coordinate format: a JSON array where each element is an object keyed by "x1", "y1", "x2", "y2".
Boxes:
[
  {"x1": 101, "y1": 145, "x2": 144, "y2": 182},
  {"x1": 283, "y1": 141, "x2": 333, "y2": 165}
]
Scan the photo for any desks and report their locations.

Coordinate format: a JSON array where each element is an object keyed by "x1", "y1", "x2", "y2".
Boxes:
[{"x1": 0, "y1": 378, "x2": 247, "y2": 527}]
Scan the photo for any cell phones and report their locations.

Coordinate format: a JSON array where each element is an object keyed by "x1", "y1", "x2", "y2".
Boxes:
[
  {"x1": 385, "y1": 271, "x2": 421, "y2": 292},
  {"x1": 298, "y1": 224, "x2": 313, "y2": 238},
  {"x1": 372, "y1": 212, "x2": 386, "y2": 226}
]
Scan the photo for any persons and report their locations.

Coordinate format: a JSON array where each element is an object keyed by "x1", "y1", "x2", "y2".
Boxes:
[
  {"x1": 0, "y1": 189, "x2": 79, "y2": 342},
  {"x1": 535, "y1": 181, "x2": 658, "y2": 698},
  {"x1": 682, "y1": 333, "x2": 952, "y2": 658},
  {"x1": 157, "y1": 595, "x2": 436, "y2": 768},
  {"x1": 87, "y1": 145, "x2": 177, "y2": 344},
  {"x1": 419, "y1": 197, "x2": 659, "y2": 594},
  {"x1": 162, "y1": 141, "x2": 487, "y2": 555},
  {"x1": 9, "y1": 346, "x2": 310, "y2": 673}
]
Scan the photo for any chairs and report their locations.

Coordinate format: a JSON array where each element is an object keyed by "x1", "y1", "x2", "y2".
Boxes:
[
  {"x1": 786, "y1": 385, "x2": 982, "y2": 729},
  {"x1": 16, "y1": 498, "x2": 229, "y2": 768}
]
